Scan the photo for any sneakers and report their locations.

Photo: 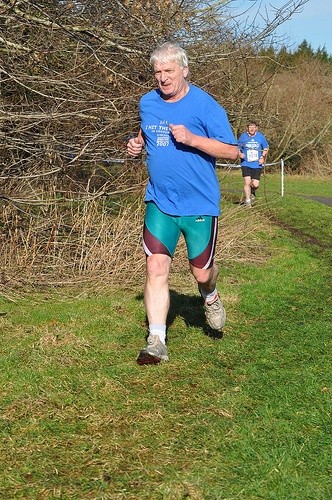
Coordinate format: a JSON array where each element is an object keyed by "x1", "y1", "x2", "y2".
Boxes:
[
  {"x1": 200, "y1": 292, "x2": 227, "y2": 330},
  {"x1": 138, "y1": 334, "x2": 170, "y2": 364}
]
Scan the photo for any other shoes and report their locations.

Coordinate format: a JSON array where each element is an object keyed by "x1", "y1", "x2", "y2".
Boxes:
[
  {"x1": 246, "y1": 201, "x2": 252, "y2": 208},
  {"x1": 251, "y1": 191, "x2": 256, "y2": 200}
]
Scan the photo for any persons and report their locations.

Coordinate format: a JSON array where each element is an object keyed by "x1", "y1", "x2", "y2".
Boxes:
[
  {"x1": 127, "y1": 41, "x2": 240, "y2": 363},
  {"x1": 238, "y1": 121, "x2": 269, "y2": 207}
]
